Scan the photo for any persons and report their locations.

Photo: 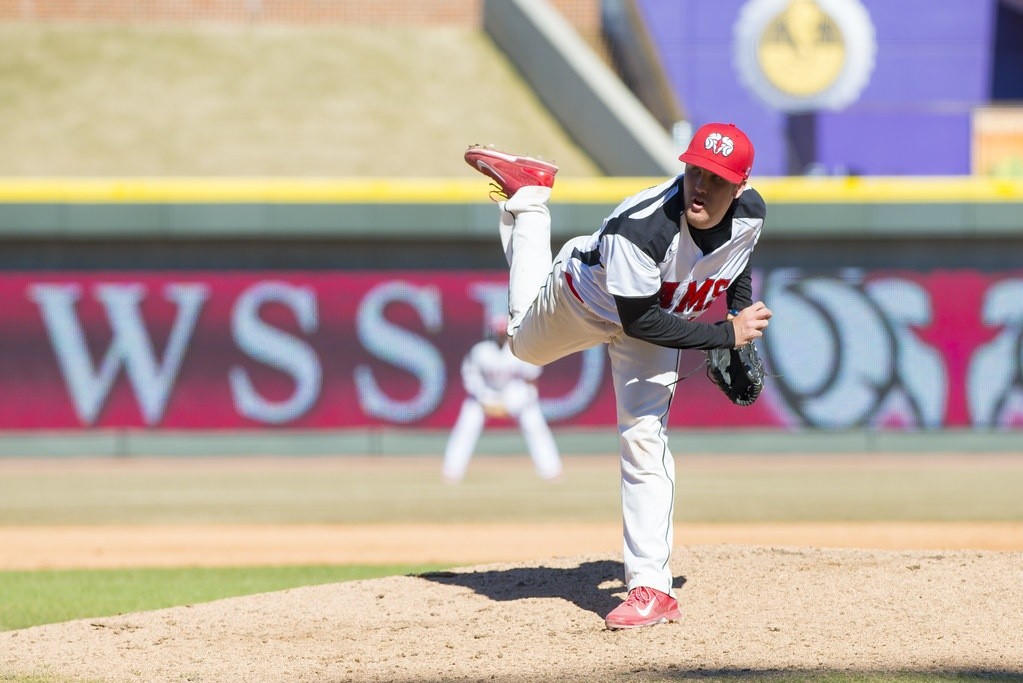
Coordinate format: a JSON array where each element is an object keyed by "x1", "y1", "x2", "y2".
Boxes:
[
  {"x1": 445, "y1": 315, "x2": 563, "y2": 480},
  {"x1": 464, "y1": 121, "x2": 772, "y2": 628}
]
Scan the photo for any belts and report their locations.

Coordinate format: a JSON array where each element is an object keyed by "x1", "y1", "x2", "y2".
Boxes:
[{"x1": 565, "y1": 272, "x2": 585, "y2": 303}]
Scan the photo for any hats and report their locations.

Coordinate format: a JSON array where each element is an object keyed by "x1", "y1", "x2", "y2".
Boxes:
[{"x1": 678, "y1": 122, "x2": 755, "y2": 185}]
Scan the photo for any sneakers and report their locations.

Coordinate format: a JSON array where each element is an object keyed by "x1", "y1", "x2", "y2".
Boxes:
[
  {"x1": 464, "y1": 143, "x2": 559, "y2": 203},
  {"x1": 604, "y1": 586, "x2": 681, "y2": 630}
]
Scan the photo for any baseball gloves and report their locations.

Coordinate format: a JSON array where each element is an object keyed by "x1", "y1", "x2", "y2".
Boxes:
[{"x1": 708, "y1": 318, "x2": 765, "y2": 406}]
